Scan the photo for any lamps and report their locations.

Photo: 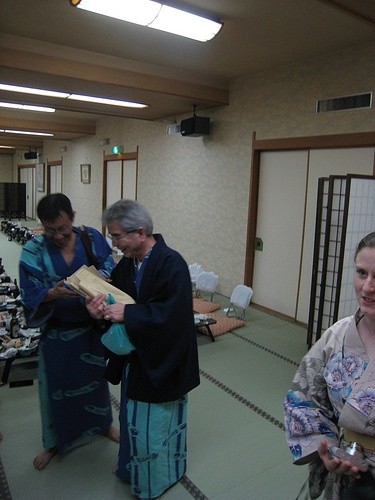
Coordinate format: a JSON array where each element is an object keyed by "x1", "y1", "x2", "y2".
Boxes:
[
  {"x1": 0, "y1": 128, "x2": 55, "y2": 138},
  {"x1": 1, "y1": 144, "x2": 14, "y2": 151},
  {"x1": 0, "y1": 82, "x2": 149, "y2": 110},
  {"x1": 0, "y1": 101, "x2": 56, "y2": 114}
]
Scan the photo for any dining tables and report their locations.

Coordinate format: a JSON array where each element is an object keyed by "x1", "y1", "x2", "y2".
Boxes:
[
  {"x1": 0, "y1": 262, "x2": 39, "y2": 388},
  {"x1": 193, "y1": 311, "x2": 218, "y2": 343}
]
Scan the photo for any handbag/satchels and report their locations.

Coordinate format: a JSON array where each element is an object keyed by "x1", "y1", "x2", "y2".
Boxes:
[{"x1": 104, "y1": 353, "x2": 127, "y2": 385}]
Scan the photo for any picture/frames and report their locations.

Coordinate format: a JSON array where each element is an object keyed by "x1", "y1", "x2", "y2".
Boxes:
[
  {"x1": 80, "y1": 164, "x2": 90, "y2": 184},
  {"x1": 35, "y1": 162, "x2": 44, "y2": 191}
]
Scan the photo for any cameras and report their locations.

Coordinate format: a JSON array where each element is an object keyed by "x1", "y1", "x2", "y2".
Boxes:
[{"x1": 331, "y1": 441, "x2": 369, "y2": 472}]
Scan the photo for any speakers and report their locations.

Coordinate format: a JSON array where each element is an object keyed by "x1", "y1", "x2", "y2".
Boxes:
[
  {"x1": 181, "y1": 117, "x2": 210, "y2": 136},
  {"x1": 24, "y1": 152, "x2": 37, "y2": 159}
]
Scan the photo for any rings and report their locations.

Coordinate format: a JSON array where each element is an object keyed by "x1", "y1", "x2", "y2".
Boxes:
[{"x1": 104, "y1": 314, "x2": 110, "y2": 320}]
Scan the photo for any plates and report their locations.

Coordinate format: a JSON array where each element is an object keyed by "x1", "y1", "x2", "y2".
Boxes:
[{"x1": 0, "y1": 327, "x2": 7, "y2": 335}]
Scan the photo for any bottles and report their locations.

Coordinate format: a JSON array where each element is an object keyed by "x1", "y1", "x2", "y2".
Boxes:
[{"x1": 10, "y1": 311, "x2": 18, "y2": 338}]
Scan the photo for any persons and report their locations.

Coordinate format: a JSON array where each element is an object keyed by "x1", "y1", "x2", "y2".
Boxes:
[
  {"x1": 84, "y1": 199, "x2": 201, "y2": 500},
  {"x1": 18, "y1": 193, "x2": 121, "y2": 471},
  {"x1": 284, "y1": 232, "x2": 375, "y2": 500}
]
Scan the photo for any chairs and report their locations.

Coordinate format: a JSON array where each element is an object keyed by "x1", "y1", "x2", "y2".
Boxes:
[
  {"x1": 202, "y1": 285, "x2": 254, "y2": 336},
  {"x1": 193, "y1": 271, "x2": 221, "y2": 314}
]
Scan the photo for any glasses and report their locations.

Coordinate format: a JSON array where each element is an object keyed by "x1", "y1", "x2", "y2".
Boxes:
[{"x1": 107, "y1": 229, "x2": 137, "y2": 240}]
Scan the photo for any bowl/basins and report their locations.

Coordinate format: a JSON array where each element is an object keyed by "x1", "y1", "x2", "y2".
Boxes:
[{"x1": 2, "y1": 340, "x2": 37, "y2": 356}]
